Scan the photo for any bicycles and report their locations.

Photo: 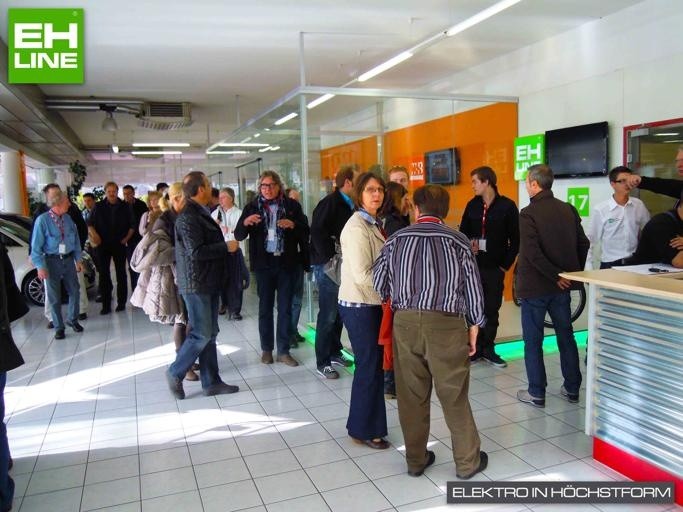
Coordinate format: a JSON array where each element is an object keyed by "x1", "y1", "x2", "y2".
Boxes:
[{"x1": 511, "y1": 252, "x2": 589, "y2": 331}]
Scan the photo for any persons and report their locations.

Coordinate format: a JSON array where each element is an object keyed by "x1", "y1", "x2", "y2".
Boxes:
[
  {"x1": 512, "y1": 164, "x2": 589, "y2": 406},
  {"x1": 371, "y1": 182, "x2": 487, "y2": 479},
  {"x1": 283, "y1": 188, "x2": 310, "y2": 348},
  {"x1": 28, "y1": 180, "x2": 245, "y2": 339},
  {"x1": 626, "y1": 145, "x2": 683, "y2": 200},
  {"x1": 309, "y1": 166, "x2": 360, "y2": 380},
  {"x1": 336, "y1": 172, "x2": 389, "y2": 450},
  {"x1": 0, "y1": 237, "x2": 30, "y2": 511},
  {"x1": 627, "y1": 192, "x2": 682, "y2": 269},
  {"x1": 374, "y1": 181, "x2": 413, "y2": 238},
  {"x1": 166, "y1": 170, "x2": 239, "y2": 399},
  {"x1": 233, "y1": 170, "x2": 301, "y2": 367},
  {"x1": 150, "y1": 181, "x2": 199, "y2": 381},
  {"x1": 584, "y1": 166, "x2": 650, "y2": 272},
  {"x1": 382, "y1": 165, "x2": 410, "y2": 194},
  {"x1": 458, "y1": 166, "x2": 519, "y2": 368}
]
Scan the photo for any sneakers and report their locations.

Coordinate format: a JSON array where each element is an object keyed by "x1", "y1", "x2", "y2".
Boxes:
[
  {"x1": 331, "y1": 353, "x2": 354, "y2": 368},
  {"x1": 516, "y1": 389, "x2": 545, "y2": 408},
  {"x1": 559, "y1": 384, "x2": 579, "y2": 403},
  {"x1": 218, "y1": 304, "x2": 242, "y2": 321},
  {"x1": 288, "y1": 334, "x2": 305, "y2": 349},
  {"x1": 165, "y1": 370, "x2": 186, "y2": 400},
  {"x1": 468, "y1": 346, "x2": 507, "y2": 368},
  {"x1": 275, "y1": 352, "x2": 299, "y2": 367},
  {"x1": 260, "y1": 349, "x2": 274, "y2": 364},
  {"x1": 202, "y1": 382, "x2": 240, "y2": 396},
  {"x1": 315, "y1": 363, "x2": 340, "y2": 380}
]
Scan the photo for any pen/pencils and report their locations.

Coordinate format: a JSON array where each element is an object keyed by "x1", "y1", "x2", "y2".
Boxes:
[{"x1": 649, "y1": 268, "x2": 669, "y2": 272}]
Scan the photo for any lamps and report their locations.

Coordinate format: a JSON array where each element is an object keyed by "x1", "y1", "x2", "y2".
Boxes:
[{"x1": 99, "y1": 104, "x2": 120, "y2": 133}]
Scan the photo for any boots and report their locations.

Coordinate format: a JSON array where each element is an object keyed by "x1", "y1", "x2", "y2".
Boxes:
[{"x1": 173, "y1": 323, "x2": 200, "y2": 381}]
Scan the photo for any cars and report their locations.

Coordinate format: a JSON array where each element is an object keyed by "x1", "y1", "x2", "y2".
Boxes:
[{"x1": 0, "y1": 208, "x2": 94, "y2": 307}]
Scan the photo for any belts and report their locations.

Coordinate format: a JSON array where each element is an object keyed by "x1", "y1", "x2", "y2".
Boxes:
[
  {"x1": 423, "y1": 308, "x2": 464, "y2": 317},
  {"x1": 612, "y1": 257, "x2": 631, "y2": 265}
]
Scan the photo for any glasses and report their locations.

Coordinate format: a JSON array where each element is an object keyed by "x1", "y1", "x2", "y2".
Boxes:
[
  {"x1": 361, "y1": 185, "x2": 385, "y2": 194},
  {"x1": 612, "y1": 179, "x2": 626, "y2": 184},
  {"x1": 260, "y1": 182, "x2": 277, "y2": 189}
]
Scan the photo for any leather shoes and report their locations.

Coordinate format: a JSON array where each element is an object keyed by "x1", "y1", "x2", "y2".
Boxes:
[
  {"x1": 455, "y1": 450, "x2": 488, "y2": 480},
  {"x1": 407, "y1": 450, "x2": 435, "y2": 477},
  {"x1": 46, "y1": 293, "x2": 126, "y2": 340},
  {"x1": 351, "y1": 435, "x2": 391, "y2": 450}
]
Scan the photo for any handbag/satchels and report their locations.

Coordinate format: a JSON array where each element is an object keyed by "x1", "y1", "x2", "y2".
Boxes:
[{"x1": 321, "y1": 238, "x2": 344, "y2": 288}]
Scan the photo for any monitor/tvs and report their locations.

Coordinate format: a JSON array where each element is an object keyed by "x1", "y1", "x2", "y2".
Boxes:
[
  {"x1": 544, "y1": 121, "x2": 609, "y2": 179},
  {"x1": 425, "y1": 148, "x2": 460, "y2": 186}
]
